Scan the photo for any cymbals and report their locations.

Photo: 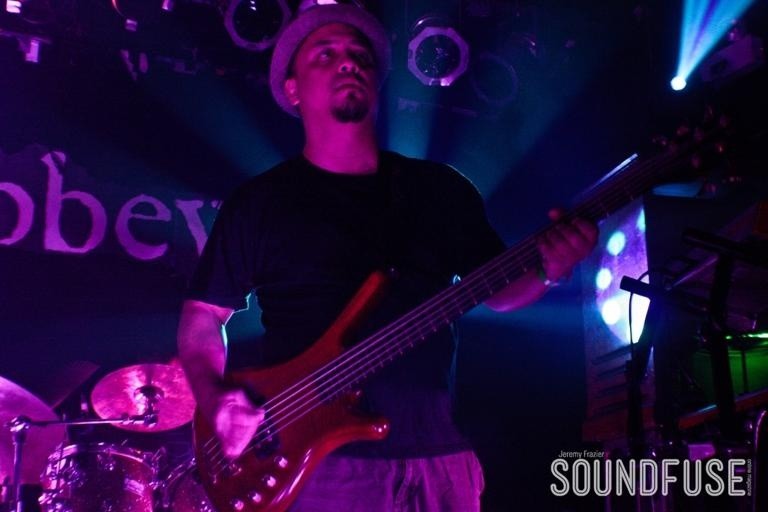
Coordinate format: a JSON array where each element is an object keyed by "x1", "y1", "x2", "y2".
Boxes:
[
  {"x1": 0, "y1": 375, "x2": 69, "y2": 486},
  {"x1": 91, "y1": 364, "x2": 196, "y2": 434}
]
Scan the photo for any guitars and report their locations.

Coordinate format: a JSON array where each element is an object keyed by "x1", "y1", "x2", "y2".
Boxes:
[{"x1": 196, "y1": 104, "x2": 731, "y2": 510}]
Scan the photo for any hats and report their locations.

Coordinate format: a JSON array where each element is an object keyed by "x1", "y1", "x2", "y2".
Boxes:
[{"x1": 269, "y1": 0, "x2": 395, "y2": 120}]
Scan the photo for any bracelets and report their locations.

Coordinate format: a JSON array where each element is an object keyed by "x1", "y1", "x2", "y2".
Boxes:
[{"x1": 532, "y1": 262, "x2": 559, "y2": 290}]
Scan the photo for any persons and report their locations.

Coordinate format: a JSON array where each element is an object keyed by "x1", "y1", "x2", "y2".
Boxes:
[{"x1": 177, "y1": 1, "x2": 601, "y2": 512}]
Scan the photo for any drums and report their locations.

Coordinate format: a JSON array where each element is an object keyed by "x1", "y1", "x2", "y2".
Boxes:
[
  {"x1": 163, "y1": 460, "x2": 217, "y2": 511},
  {"x1": 41, "y1": 441, "x2": 158, "y2": 512}
]
